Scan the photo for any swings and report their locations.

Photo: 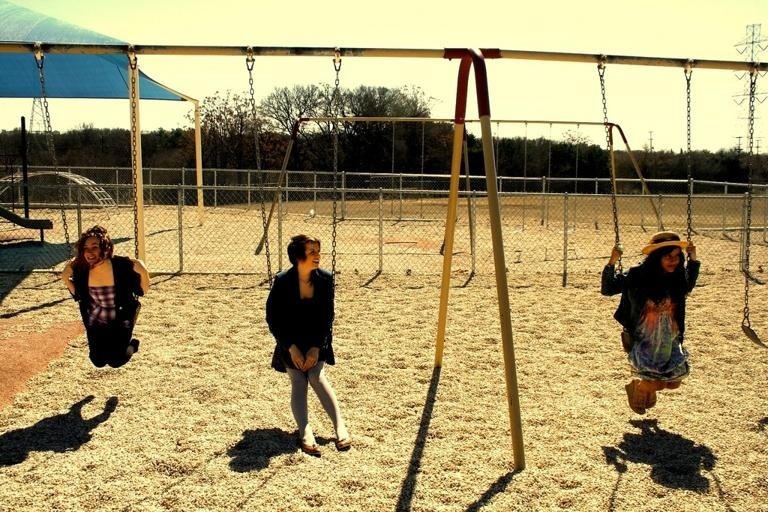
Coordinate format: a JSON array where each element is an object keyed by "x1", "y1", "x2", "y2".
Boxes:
[
  {"x1": 546, "y1": 121, "x2": 580, "y2": 236},
  {"x1": 596, "y1": 57, "x2": 692, "y2": 351},
  {"x1": 494, "y1": 119, "x2": 529, "y2": 238},
  {"x1": 244, "y1": 46, "x2": 342, "y2": 363},
  {"x1": 32, "y1": 40, "x2": 141, "y2": 330},
  {"x1": 739, "y1": 62, "x2": 768, "y2": 353},
  {"x1": 388, "y1": 116, "x2": 425, "y2": 224}
]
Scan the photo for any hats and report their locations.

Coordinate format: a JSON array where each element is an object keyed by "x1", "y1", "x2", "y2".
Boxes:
[{"x1": 641, "y1": 230, "x2": 691, "y2": 256}]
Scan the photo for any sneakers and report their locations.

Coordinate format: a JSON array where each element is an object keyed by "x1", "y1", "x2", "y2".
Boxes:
[
  {"x1": 300, "y1": 435, "x2": 321, "y2": 456},
  {"x1": 335, "y1": 433, "x2": 352, "y2": 449},
  {"x1": 624, "y1": 379, "x2": 658, "y2": 413},
  {"x1": 130, "y1": 338, "x2": 139, "y2": 353}
]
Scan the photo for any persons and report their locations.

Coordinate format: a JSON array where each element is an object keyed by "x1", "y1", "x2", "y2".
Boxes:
[
  {"x1": 60, "y1": 224, "x2": 151, "y2": 369},
  {"x1": 264, "y1": 233, "x2": 351, "y2": 456},
  {"x1": 599, "y1": 231, "x2": 700, "y2": 416}
]
